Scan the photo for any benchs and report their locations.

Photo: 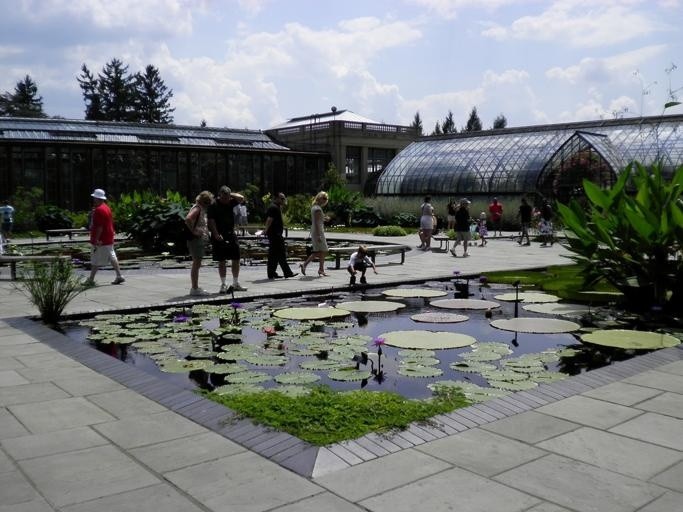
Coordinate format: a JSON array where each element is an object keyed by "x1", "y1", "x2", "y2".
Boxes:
[
  {"x1": 239, "y1": 225, "x2": 288, "y2": 237},
  {"x1": 328, "y1": 244, "x2": 408, "y2": 270},
  {"x1": 0, "y1": 255, "x2": 71, "y2": 281},
  {"x1": 46, "y1": 229, "x2": 88, "y2": 241},
  {"x1": 432, "y1": 234, "x2": 450, "y2": 254}
]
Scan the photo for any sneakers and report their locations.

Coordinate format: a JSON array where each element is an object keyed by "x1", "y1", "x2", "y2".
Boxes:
[
  {"x1": 111, "y1": 275, "x2": 125, "y2": 285},
  {"x1": 349, "y1": 276, "x2": 368, "y2": 285},
  {"x1": 80, "y1": 277, "x2": 96, "y2": 288},
  {"x1": 463, "y1": 253, "x2": 470, "y2": 256},
  {"x1": 190, "y1": 282, "x2": 249, "y2": 296},
  {"x1": 450, "y1": 248, "x2": 457, "y2": 257}
]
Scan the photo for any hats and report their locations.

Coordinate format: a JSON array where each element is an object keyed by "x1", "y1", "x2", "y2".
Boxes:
[
  {"x1": 460, "y1": 198, "x2": 471, "y2": 204},
  {"x1": 90, "y1": 188, "x2": 107, "y2": 201}
]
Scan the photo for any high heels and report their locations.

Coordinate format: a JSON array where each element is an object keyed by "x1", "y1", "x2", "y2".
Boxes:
[
  {"x1": 299, "y1": 263, "x2": 307, "y2": 277},
  {"x1": 317, "y1": 270, "x2": 330, "y2": 277}
]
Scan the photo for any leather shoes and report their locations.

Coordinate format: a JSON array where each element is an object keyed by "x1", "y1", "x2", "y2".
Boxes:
[{"x1": 267, "y1": 273, "x2": 299, "y2": 280}]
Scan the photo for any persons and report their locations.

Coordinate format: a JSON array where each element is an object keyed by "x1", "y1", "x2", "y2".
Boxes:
[
  {"x1": 183, "y1": 189, "x2": 214, "y2": 297},
  {"x1": 208, "y1": 185, "x2": 248, "y2": 293},
  {"x1": 477, "y1": 211, "x2": 488, "y2": 248},
  {"x1": 488, "y1": 197, "x2": 504, "y2": 237},
  {"x1": 297, "y1": 190, "x2": 332, "y2": 277},
  {"x1": 515, "y1": 197, "x2": 556, "y2": 246},
  {"x1": 260, "y1": 192, "x2": 299, "y2": 279},
  {"x1": 0, "y1": 200, "x2": 15, "y2": 237},
  {"x1": 79, "y1": 187, "x2": 126, "y2": 288},
  {"x1": 347, "y1": 244, "x2": 379, "y2": 287},
  {"x1": 416, "y1": 195, "x2": 475, "y2": 258}
]
